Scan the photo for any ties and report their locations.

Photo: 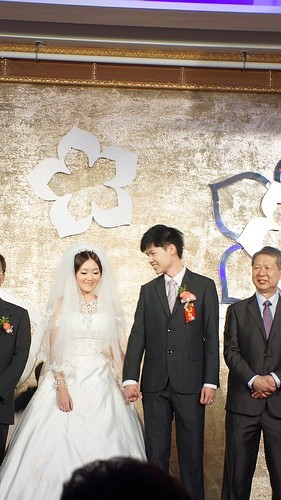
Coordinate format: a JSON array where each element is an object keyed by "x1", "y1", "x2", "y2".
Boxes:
[
  {"x1": 161, "y1": 279, "x2": 181, "y2": 310},
  {"x1": 258, "y1": 304, "x2": 278, "y2": 337}
]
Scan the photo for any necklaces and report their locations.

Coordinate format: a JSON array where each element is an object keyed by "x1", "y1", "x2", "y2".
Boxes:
[{"x1": 78, "y1": 299, "x2": 99, "y2": 316}]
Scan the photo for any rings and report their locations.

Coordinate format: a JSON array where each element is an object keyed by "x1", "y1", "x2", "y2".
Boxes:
[{"x1": 209, "y1": 399, "x2": 214, "y2": 405}]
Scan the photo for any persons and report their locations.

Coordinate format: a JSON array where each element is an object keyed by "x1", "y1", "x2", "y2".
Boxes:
[
  {"x1": 220, "y1": 248, "x2": 281, "y2": 500},
  {"x1": 0, "y1": 245, "x2": 147, "y2": 500},
  {"x1": 0, "y1": 251, "x2": 32, "y2": 462},
  {"x1": 120, "y1": 225, "x2": 219, "y2": 500},
  {"x1": 60, "y1": 457, "x2": 192, "y2": 499}
]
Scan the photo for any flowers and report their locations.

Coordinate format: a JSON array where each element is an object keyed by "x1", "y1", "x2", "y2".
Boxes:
[
  {"x1": 177, "y1": 284, "x2": 197, "y2": 323},
  {"x1": 0, "y1": 316, "x2": 13, "y2": 333}
]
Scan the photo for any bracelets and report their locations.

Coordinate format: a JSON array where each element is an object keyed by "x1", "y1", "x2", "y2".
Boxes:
[{"x1": 52, "y1": 375, "x2": 69, "y2": 390}]
